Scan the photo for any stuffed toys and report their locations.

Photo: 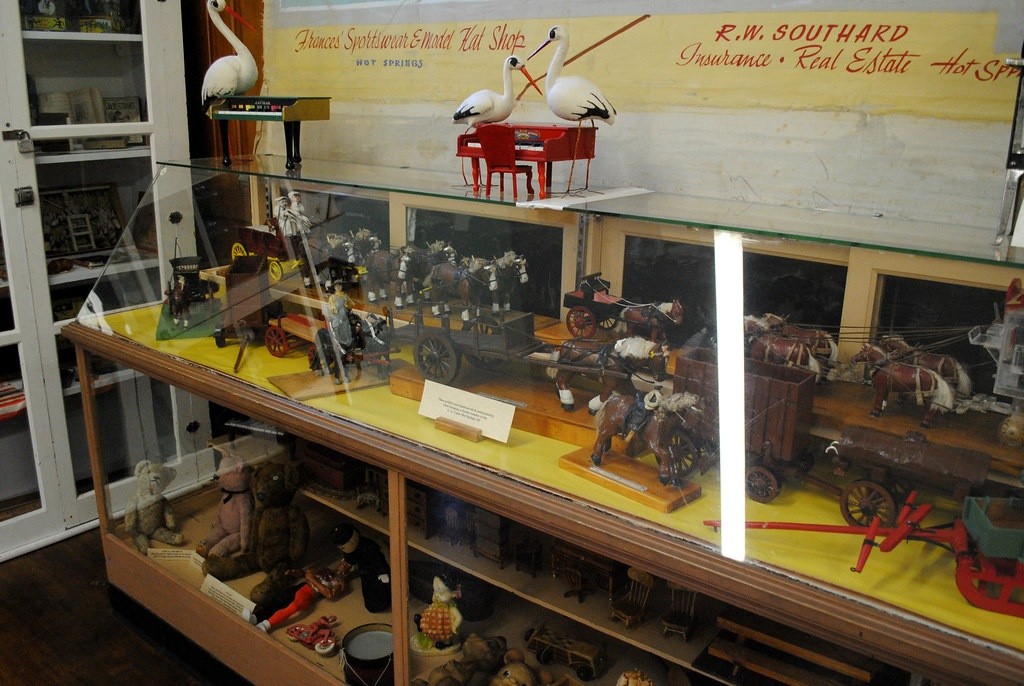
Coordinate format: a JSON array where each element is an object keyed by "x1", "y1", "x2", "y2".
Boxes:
[
  {"x1": 124, "y1": 459, "x2": 184, "y2": 555},
  {"x1": 196, "y1": 451, "x2": 310, "y2": 604}
]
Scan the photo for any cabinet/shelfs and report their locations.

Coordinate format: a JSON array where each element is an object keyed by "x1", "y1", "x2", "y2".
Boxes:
[
  {"x1": 1, "y1": 0, "x2": 281, "y2": 564},
  {"x1": 61, "y1": 155, "x2": 1023, "y2": 686}
]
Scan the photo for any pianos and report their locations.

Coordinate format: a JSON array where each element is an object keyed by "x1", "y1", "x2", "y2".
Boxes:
[
  {"x1": 455, "y1": 120, "x2": 599, "y2": 200},
  {"x1": 204, "y1": 95, "x2": 332, "y2": 170}
]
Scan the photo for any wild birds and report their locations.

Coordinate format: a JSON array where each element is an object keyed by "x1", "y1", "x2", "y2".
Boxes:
[
  {"x1": 452, "y1": 55, "x2": 544, "y2": 189},
  {"x1": 527, "y1": 25, "x2": 618, "y2": 199},
  {"x1": 199, "y1": 0, "x2": 259, "y2": 166}
]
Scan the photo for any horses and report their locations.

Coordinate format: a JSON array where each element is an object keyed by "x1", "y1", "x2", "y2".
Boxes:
[{"x1": 265, "y1": 216, "x2": 976, "y2": 492}]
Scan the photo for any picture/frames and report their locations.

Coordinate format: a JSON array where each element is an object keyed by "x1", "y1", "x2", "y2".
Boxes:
[{"x1": 40, "y1": 181, "x2": 135, "y2": 257}]
